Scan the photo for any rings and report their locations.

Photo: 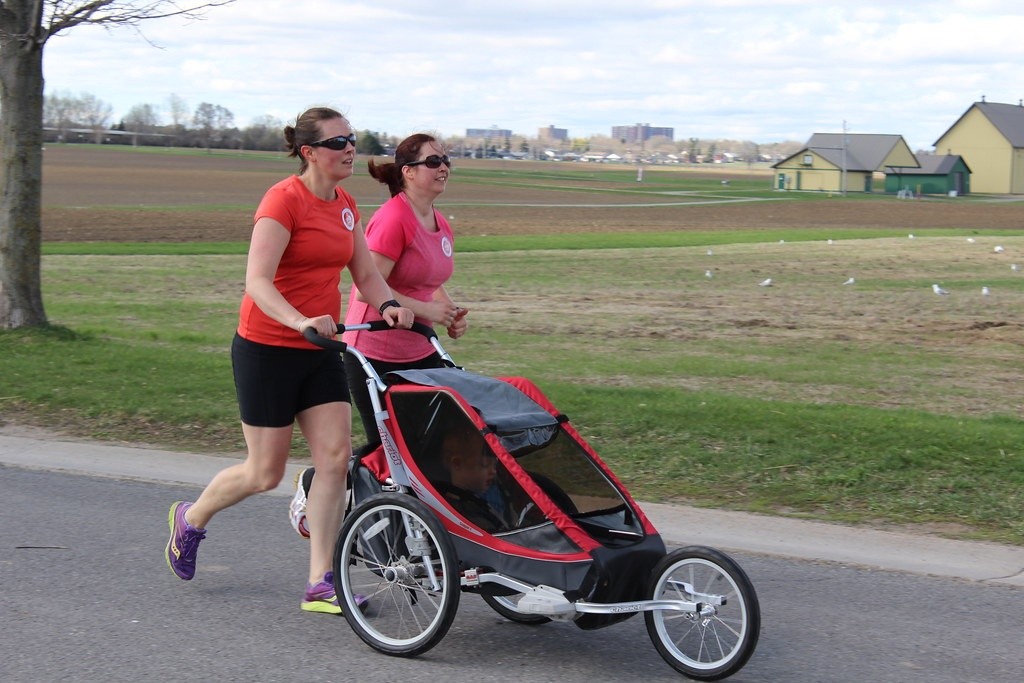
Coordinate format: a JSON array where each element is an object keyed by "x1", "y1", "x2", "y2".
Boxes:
[{"x1": 410, "y1": 322, "x2": 413, "y2": 325}]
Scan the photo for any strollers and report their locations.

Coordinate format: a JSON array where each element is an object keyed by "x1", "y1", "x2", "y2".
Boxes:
[{"x1": 295, "y1": 319, "x2": 766, "y2": 680}]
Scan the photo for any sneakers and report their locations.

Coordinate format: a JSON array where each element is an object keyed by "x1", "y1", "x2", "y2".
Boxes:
[
  {"x1": 289, "y1": 467, "x2": 316, "y2": 541},
  {"x1": 300, "y1": 571, "x2": 368, "y2": 614},
  {"x1": 165, "y1": 501, "x2": 206, "y2": 581}
]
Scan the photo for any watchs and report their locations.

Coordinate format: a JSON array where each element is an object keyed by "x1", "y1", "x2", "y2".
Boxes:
[{"x1": 379, "y1": 300, "x2": 400, "y2": 316}]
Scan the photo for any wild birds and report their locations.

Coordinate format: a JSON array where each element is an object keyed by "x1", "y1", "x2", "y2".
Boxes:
[{"x1": 704, "y1": 231, "x2": 1018, "y2": 298}]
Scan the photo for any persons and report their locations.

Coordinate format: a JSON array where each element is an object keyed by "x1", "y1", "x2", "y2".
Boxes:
[
  {"x1": 164, "y1": 108, "x2": 369, "y2": 614},
  {"x1": 285, "y1": 132, "x2": 469, "y2": 538},
  {"x1": 442, "y1": 426, "x2": 530, "y2": 536}
]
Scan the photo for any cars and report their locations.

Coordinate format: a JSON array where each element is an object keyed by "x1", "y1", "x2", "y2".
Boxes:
[{"x1": 449, "y1": 144, "x2": 681, "y2": 167}]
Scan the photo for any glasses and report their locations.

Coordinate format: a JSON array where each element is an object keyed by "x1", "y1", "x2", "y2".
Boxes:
[
  {"x1": 407, "y1": 155, "x2": 451, "y2": 169},
  {"x1": 310, "y1": 133, "x2": 356, "y2": 150}
]
